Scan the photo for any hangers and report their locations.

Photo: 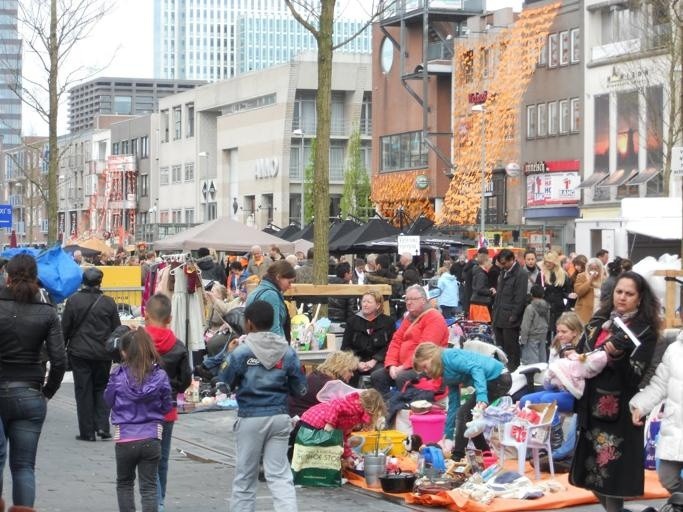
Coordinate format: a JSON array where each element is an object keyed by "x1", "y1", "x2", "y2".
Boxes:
[{"x1": 147, "y1": 254, "x2": 202, "y2": 276}]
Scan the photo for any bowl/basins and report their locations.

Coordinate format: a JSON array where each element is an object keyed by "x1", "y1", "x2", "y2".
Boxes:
[{"x1": 363, "y1": 455, "x2": 386, "y2": 489}]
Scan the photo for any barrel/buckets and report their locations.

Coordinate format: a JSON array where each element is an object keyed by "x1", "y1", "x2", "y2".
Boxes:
[{"x1": 408, "y1": 410, "x2": 447, "y2": 445}]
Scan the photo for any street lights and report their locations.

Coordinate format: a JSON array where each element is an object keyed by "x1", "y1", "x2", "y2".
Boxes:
[
  {"x1": 469, "y1": 103, "x2": 486, "y2": 240},
  {"x1": 293, "y1": 129, "x2": 304, "y2": 231},
  {"x1": 198, "y1": 151, "x2": 209, "y2": 222},
  {"x1": 58, "y1": 175, "x2": 67, "y2": 241},
  {"x1": 15, "y1": 183, "x2": 22, "y2": 241}
]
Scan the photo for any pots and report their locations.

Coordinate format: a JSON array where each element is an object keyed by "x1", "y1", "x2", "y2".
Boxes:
[{"x1": 378, "y1": 467, "x2": 418, "y2": 492}]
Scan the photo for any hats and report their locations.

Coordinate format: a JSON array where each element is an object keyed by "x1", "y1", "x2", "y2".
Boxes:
[{"x1": 83, "y1": 268, "x2": 103, "y2": 285}]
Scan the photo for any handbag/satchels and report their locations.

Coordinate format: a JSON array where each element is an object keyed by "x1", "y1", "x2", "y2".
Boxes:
[
  {"x1": 65, "y1": 347, "x2": 72, "y2": 371},
  {"x1": 222, "y1": 306, "x2": 249, "y2": 335},
  {"x1": 290, "y1": 426, "x2": 344, "y2": 487}
]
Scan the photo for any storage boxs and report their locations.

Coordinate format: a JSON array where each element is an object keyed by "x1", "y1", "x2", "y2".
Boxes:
[{"x1": 351, "y1": 430, "x2": 407, "y2": 460}]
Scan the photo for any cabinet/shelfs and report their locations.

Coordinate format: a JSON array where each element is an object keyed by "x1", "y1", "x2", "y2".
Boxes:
[{"x1": 247, "y1": 284, "x2": 392, "y2": 376}]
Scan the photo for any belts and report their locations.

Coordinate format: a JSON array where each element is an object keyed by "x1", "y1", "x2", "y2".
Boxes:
[{"x1": 0, "y1": 381, "x2": 43, "y2": 389}]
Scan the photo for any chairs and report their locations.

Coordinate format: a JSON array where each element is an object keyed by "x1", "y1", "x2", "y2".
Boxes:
[{"x1": 501, "y1": 403, "x2": 558, "y2": 480}]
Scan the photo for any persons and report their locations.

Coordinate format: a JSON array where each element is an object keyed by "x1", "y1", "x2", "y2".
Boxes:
[
  {"x1": 340, "y1": 292, "x2": 394, "y2": 382},
  {"x1": 520, "y1": 314, "x2": 585, "y2": 449},
  {"x1": 241, "y1": 259, "x2": 295, "y2": 346},
  {"x1": 371, "y1": 283, "x2": 450, "y2": 399},
  {"x1": 1, "y1": 243, "x2": 635, "y2": 368},
  {"x1": 104, "y1": 293, "x2": 192, "y2": 511},
  {"x1": 289, "y1": 351, "x2": 360, "y2": 417},
  {"x1": 630, "y1": 330, "x2": 683, "y2": 510},
  {"x1": 289, "y1": 390, "x2": 389, "y2": 486},
  {"x1": 60, "y1": 267, "x2": 120, "y2": 443},
  {"x1": 0, "y1": 253, "x2": 67, "y2": 512},
  {"x1": 413, "y1": 342, "x2": 512, "y2": 465},
  {"x1": 569, "y1": 270, "x2": 659, "y2": 512},
  {"x1": 222, "y1": 301, "x2": 309, "y2": 512},
  {"x1": 103, "y1": 326, "x2": 173, "y2": 512}
]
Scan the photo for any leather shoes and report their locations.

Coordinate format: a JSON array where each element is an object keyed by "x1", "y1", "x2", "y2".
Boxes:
[
  {"x1": 97, "y1": 429, "x2": 113, "y2": 437},
  {"x1": 76, "y1": 435, "x2": 96, "y2": 441}
]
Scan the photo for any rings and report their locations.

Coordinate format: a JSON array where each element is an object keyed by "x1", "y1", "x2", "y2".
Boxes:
[{"x1": 613, "y1": 349, "x2": 617, "y2": 353}]
